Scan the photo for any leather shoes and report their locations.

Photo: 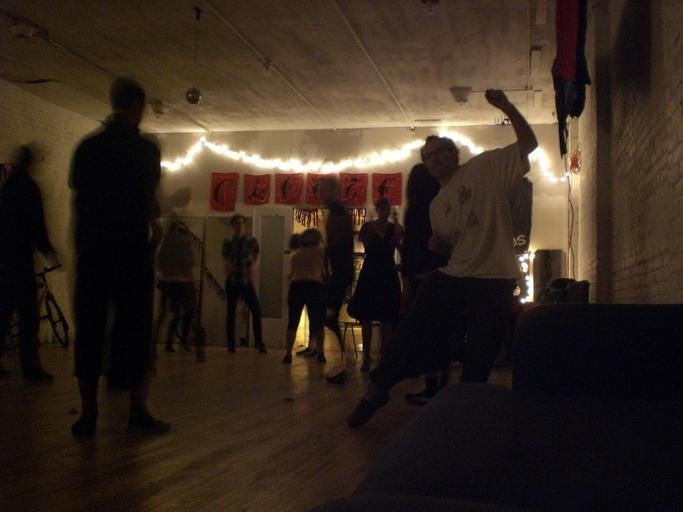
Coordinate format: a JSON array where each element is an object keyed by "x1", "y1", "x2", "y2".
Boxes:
[
  {"x1": 127, "y1": 415, "x2": 170, "y2": 435},
  {"x1": 347, "y1": 391, "x2": 391, "y2": 429},
  {"x1": 71, "y1": 416, "x2": 96, "y2": 434}
]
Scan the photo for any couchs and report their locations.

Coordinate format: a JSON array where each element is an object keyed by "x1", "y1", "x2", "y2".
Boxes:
[{"x1": 309, "y1": 302, "x2": 681, "y2": 512}]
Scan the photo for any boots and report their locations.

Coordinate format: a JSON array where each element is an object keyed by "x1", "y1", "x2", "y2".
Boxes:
[{"x1": 406, "y1": 373, "x2": 449, "y2": 408}]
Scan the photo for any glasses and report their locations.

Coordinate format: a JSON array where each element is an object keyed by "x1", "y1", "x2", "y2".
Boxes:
[{"x1": 422, "y1": 145, "x2": 450, "y2": 161}]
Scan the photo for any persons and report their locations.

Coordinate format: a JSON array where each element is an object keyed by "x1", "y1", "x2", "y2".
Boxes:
[
  {"x1": 64, "y1": 79, "x2": 174, "y2": 440},
  {"x1": 152, "y1": 213, "x2": 266, "y2": 362},
  {"x1": 1, "y1": 143, "x2": 63, "y2": 388},
  {"x1": 277, "y1": 88, "x2": 537, "y2": 428}
]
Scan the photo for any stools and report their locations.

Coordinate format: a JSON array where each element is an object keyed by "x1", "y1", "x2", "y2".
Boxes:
[{"x1": 341, "y1": 322, "x2": 378, "y2": 360}]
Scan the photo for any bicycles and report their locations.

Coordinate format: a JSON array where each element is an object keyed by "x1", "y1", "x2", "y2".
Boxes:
[{"x1": 2, "y1": 264, "x2": 69, "y2": 352}]
